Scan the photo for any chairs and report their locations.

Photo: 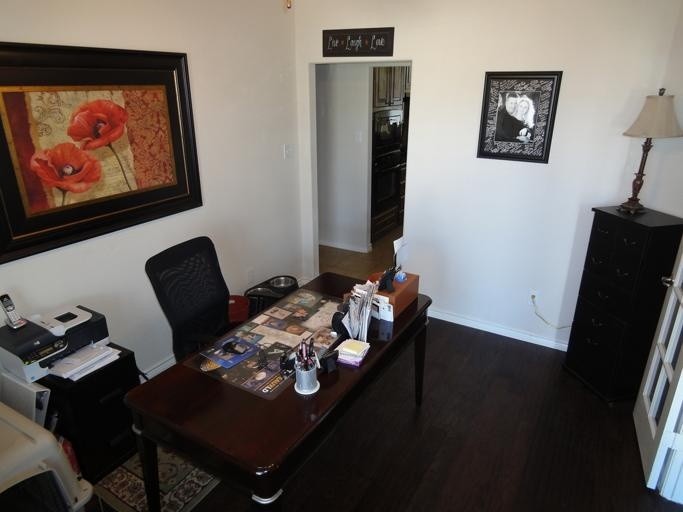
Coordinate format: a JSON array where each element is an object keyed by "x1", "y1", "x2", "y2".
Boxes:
[{"x1": 144, "y1": 236, "x2": 244, "y2": 365}]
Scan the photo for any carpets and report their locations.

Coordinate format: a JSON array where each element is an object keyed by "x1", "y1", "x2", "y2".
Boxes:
[{"x1": 93, "y1": 444, "x2": 219, "y2": 511}]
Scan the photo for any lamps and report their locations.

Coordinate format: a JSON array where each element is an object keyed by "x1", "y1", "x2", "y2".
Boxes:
[{"x1": 615, "y1": 88, "x2": 683, "y2": 216}]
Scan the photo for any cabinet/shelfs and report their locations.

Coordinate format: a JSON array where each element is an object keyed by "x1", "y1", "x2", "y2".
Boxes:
[
  {"x1": 561, "y1": 205, "x2": 683, "y2": 404},
  {"x1": 32, "y1": 341, "x2": 141, "y2": 486},
  {"x1": 370, "y1": 65, "x2": 411, "y2": 244}
]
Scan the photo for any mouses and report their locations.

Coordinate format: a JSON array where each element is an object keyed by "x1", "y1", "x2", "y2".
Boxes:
[{"x1": 223, "y1": 342, "x2": 248, "y2": 354}]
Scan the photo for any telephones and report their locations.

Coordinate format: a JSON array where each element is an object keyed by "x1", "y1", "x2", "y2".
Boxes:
[{"x1": 0, "y1": 295, "x2": 28, "y2": 330}]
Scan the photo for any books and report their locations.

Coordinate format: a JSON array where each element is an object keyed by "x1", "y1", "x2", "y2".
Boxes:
[
  {"x1": 47, "y1": 342, "x2": 122, "y2": 382},
  {"x1": 335, "y1": 356, "x2": 362, "y2": 368},
  {"x1": 337, "y1": 345, "x2": 371, "y2": 356}
]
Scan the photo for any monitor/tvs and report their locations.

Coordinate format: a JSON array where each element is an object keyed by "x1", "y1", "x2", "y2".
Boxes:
[{"x1": 372, "y1": 105, "x2": 405, "y2": 160}]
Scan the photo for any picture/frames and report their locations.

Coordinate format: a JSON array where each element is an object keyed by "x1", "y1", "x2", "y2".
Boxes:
[
  {"x1": 0, "y1": 41, "x2": 202, "y2": 265},
  {"x1": 476, "y1": 70, "x2": 563, "y2": 165},
  {"x1": 321, "y1": 27, "x2": 394, "y2": 57}
]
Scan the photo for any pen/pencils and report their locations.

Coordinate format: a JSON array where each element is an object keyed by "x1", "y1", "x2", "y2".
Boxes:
[{"x1": 296, "y1": 337, "x2": 316, "y2": 371}]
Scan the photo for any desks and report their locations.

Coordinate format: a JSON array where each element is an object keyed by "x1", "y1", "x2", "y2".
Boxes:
[{"x1": 121, "y1": 272, "x2": 431, "y2": 512}]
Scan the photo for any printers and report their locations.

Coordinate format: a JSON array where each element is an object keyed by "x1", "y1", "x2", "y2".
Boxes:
[{"x1": 0, "y1": 305, "x2": 112, "y2": 385}]
